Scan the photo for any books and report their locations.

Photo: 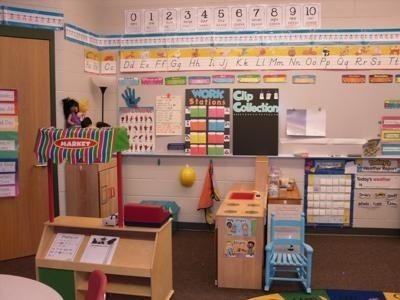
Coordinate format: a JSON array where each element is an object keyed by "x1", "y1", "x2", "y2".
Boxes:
[
  {"x1": 80, "y1": 235, "x2": 120, "y2": 265},
  {"x1": 44, "y1": 233, "x2": 85, "y2": 261}
]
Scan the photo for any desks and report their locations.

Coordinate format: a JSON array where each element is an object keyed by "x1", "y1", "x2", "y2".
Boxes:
[
  {"x1": 0, "y1": 274, "x2": 64, "y2": 300},
  {"x1": 248, "y1": 290, "x2": 400, "y2": 300}
]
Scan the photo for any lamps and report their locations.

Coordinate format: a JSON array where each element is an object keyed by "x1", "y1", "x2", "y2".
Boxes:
[{"x1": 92, "y1": 76, "x2": 116, "y2": 127}]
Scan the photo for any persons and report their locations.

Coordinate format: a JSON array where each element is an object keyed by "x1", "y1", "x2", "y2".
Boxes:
[{"x1": 63, "y1": 99, "x2": 89, "y2": 127}]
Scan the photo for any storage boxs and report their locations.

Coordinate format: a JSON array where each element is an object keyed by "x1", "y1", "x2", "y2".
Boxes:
[
  {"x1": 142, "y1": 200, "x2": 180, "y2": 230},
  {"x1": 124, "y1": 203, "x2": 169, "y2": 228}
]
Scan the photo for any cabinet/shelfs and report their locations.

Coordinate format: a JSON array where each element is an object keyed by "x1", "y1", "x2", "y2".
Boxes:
[
  {"x1": 215, "y1": 189, "x2": 267, "y2": 290},
  {"x1": 267, "y1": 183, "x2": 302, "y2": 205},
  {"x1": 35, "y1": 216, "x2": 174, "y2": 299},
  {"x1": 65, "y1": 160, "x2": 117, "y2": 218}
]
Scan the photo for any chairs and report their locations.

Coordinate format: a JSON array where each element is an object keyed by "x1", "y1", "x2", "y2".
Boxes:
[
  {"x1": 264, "y1": 212, "x2": 314, "y2": 292},
  {"x1": 85, "y1": 269, "x2": 107, "y2": 299}
]
